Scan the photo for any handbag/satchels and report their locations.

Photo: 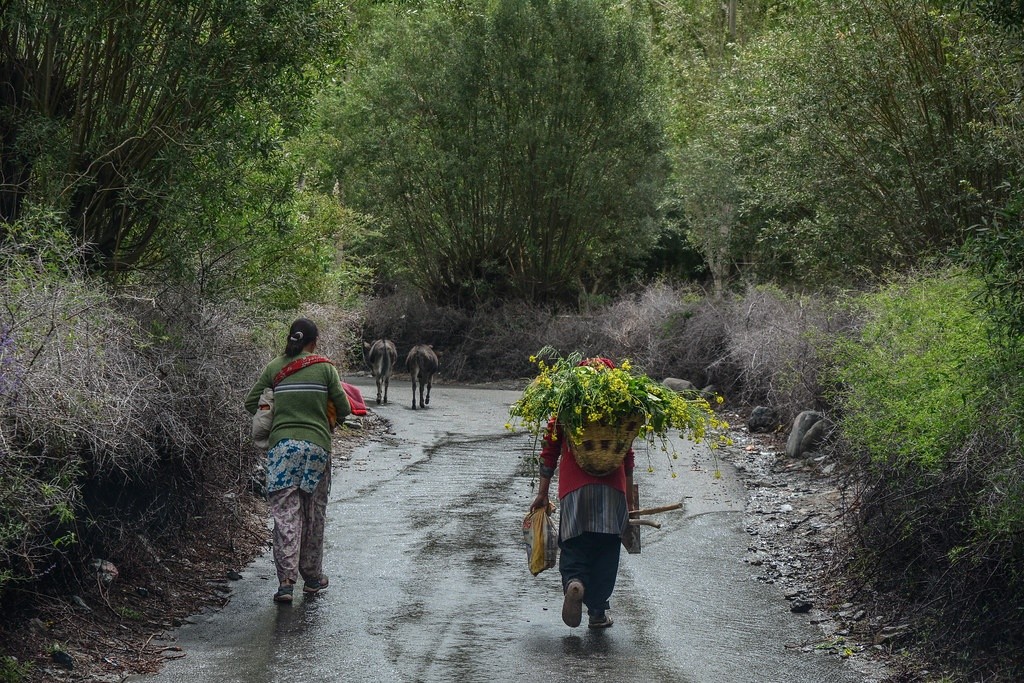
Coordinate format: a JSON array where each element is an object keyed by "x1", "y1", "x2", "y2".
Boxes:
[{"x1": 523, "y1": 502, "x2": 558, "y2": 577}]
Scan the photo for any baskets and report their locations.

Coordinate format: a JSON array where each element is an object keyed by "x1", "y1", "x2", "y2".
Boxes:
[{"x1": 564, "y1": 399, "x2": 646, "y2": 477}]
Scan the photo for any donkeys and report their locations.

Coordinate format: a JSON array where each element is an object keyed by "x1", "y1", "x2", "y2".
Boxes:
[
  {"x1": 364, "y1": 338, "x2": 397, "y2": 404},
  {"x1": 406, "y1": 344, "x2": 443, "y2": 410}
]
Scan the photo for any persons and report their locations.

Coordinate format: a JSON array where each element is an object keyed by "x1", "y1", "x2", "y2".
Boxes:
[
  {"x1": 244, "y1": 319, "x2": 350, "y2": 601},
  {"x1": 530, "y1": 359, "x2": 635, "y2": 629}
]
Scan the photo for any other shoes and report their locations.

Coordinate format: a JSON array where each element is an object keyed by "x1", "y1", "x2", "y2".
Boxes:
[
  {"x1": 273, "y1": 584, "x2": 294, "y2": 603},
  {"x1": 303, "y1": 573, "x2": 329, "y2": 592},
  {"x1": 562, "y1": 580, "x2": 584, "y2": 628},
  {"x1": 588, "y1": 613, "x2": 615, "y2": 629}
]
